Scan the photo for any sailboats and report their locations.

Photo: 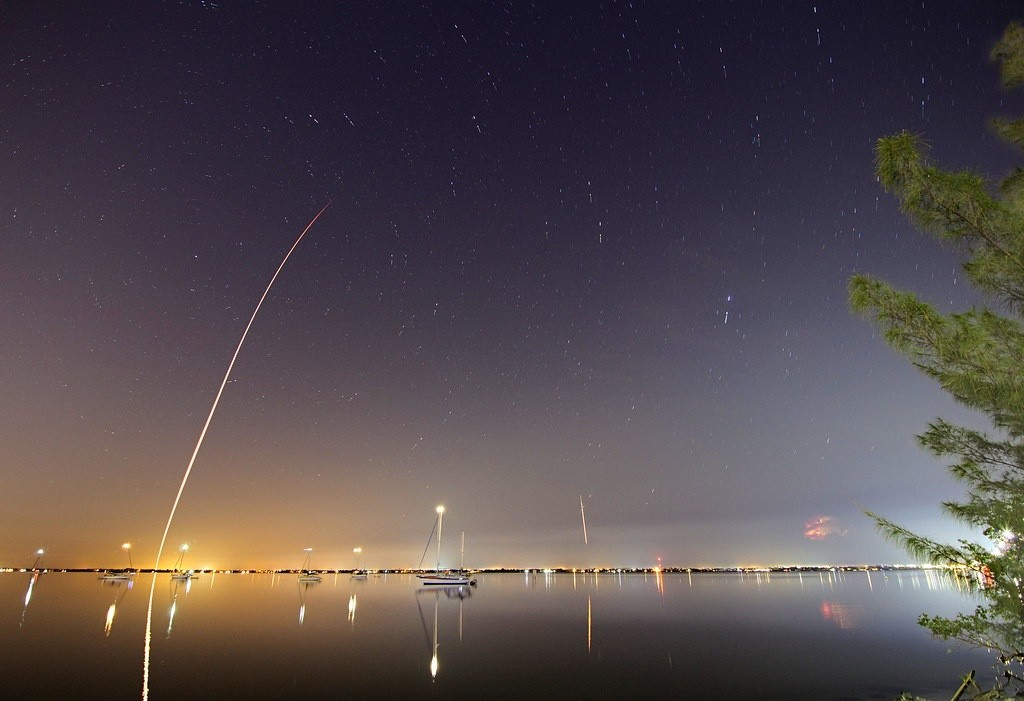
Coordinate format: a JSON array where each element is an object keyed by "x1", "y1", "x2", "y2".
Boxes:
[
  {"x1": 415, "y1": 502, "x2": 479, "y2": 587},
  {"x1": 170, "y1": 543, "x2": 198, "y2": 579},
  {"x1": 25, "y1": 548, "x2": 49, "y2": 574},
  {"x1": 351, "y1": 547, "x2": 367, "y2": 580},
  {"x1": 416, "y1": 584, "x2": 474, "y2": 683},
  {"x1": 99, "y1": 542, "x2": 136, "y2": 581},
  {"x1": 296, "y1": 546, "x2": 321, "y2": 582}
]
